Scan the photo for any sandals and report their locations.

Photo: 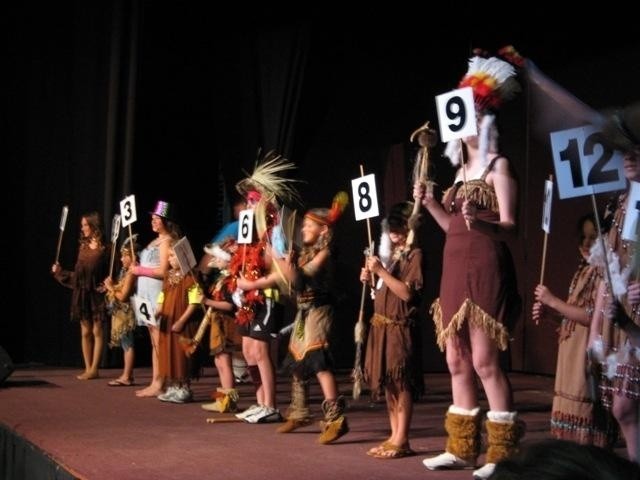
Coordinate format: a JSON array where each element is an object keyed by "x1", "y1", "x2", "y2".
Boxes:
[{"x1": 367, "y1": 439, "x2": 415, "y2": 460}]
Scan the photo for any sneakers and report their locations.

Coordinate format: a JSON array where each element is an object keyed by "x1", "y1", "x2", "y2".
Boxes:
[
  {"x1": 157, "y1": 385, "x2": 193, "y2": 404},
  {"x1": 234, "y1": 403, "x2": 282, "y2": 425}
]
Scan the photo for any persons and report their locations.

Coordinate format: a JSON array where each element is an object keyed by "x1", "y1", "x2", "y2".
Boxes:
[
  {"x1": 488, "y1": 107, "x2": 639, "y2": 480},
  {"x1": 52, "y1": 150, "x2": 349, "y2": 444},
  {"x1": 360, "y1": 200, "x2": 423, "y2": 457},
  {"x1": 414, "y1": 55, "x2": 517, "y2": 478}
]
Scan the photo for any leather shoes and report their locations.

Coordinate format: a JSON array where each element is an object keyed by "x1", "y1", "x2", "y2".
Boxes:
[{"x1": 76, "y1": 368, "x2": 100, "y2": 381}]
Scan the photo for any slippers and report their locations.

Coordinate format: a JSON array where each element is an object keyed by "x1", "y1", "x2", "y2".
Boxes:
[{"x1": 107, "y1": 376, "x2": 137, "y2": 386}]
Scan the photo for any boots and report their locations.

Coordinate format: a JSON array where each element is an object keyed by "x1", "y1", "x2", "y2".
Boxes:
[
  {"x1": 275, "y1": 379, "x2": 316, "y2": 434},
  {"x1": 200, "y1": 386, "x2": 237, "y2": 413},
  {"x1": 420, "y1": 404, "x2": 482, "y2": 471},
  {"x1": 473, "y1": 408, "x2": 523, "y2": 479},
  {"x1": 317, "y1": 395, "x2": 349, "y2": 446}
]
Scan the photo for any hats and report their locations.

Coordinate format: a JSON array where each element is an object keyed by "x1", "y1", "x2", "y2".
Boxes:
[
  {"x1": 203, "y1": 236, "x2": 242, "y2": 263},
  {"x1": 235, "y1": 175, "x2": 283, "y2": 214},
  {"x1": 456, "y1": 45, "x2": 527, "y2": 118},
  {"x1": 148, "y1": 198, "x2": 184, "y2": 223},
  {"x1": 387, "y1": 199, "x2": 425, "y2": 230}
]
